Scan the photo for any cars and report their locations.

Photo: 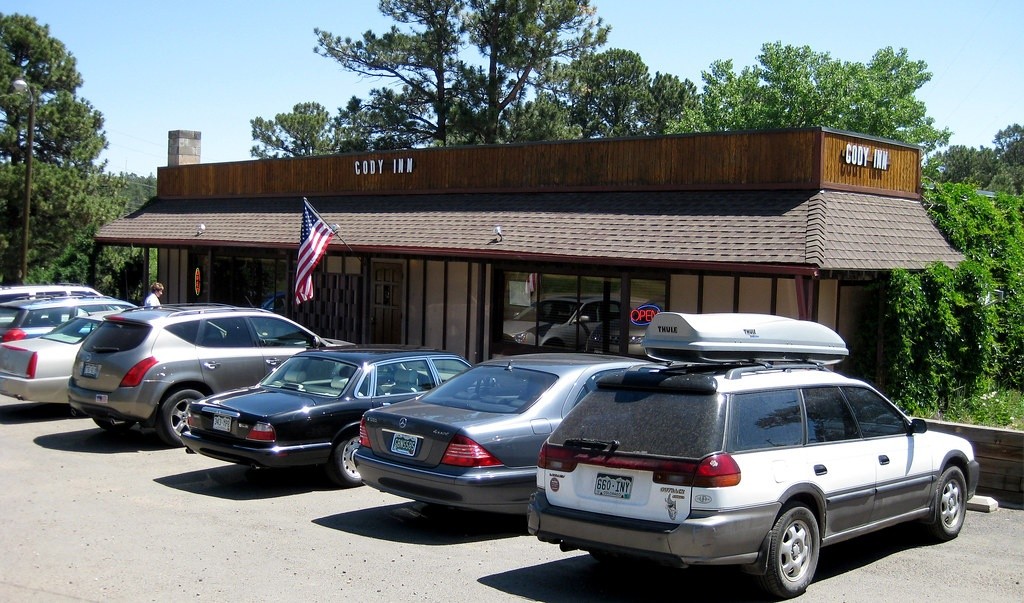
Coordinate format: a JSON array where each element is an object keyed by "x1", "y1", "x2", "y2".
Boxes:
[
  {"x1": 178, "y1": 347, "x2": 472, "y2": 487},
  {"x1": 0, "y1": 309, "x2": 228, "y2": 417},
  {"x1": 353, "y1": 352, "x2": 653, "y2": 528},
  {"x1": 503, "y1": 294, "x2": 664, "y2": 358}
]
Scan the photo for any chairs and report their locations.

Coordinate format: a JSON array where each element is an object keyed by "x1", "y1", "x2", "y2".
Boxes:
[{"x1": 390, "y1": 370, "x2": 424, "y2": 393}]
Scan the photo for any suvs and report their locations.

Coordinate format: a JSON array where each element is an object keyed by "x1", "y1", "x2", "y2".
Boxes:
[
  {"x1": 67, "y1": 303, "x2": 357, "y2": 450},
  {"x1": 0, "y1": 284, "x2": 137, "y2": 339},
  {"x1": 526, "y1": 314, "x2": 982, "y2": 600}
]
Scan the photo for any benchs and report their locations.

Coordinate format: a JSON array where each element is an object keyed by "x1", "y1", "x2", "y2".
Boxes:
[
  {"x1": 454, "y1": 390, "x2": 527, "y2": 408},
  {"x1": 273, "y1": 369, "x2": 365, "y2": 397}
]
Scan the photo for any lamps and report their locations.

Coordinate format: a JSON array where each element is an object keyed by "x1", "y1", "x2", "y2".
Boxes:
[
  {"x1": 493, "y1": 227, "x2": 503, "y2": 242},
  {"x1": 196, "y1": 224, "x2": 206, "y2": 235}
]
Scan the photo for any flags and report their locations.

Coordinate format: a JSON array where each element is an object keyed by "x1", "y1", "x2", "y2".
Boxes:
[
  {"x1": 294, "y1": 201, "x2": 335, "y2": 304},
  {"x1": 526, "y1": 273, "x2": 536, "y2": 293}
]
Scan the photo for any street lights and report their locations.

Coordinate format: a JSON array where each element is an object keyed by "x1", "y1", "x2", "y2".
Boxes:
[{"x1": 13, "y1": 77, "x2": 38, "y2": 285}]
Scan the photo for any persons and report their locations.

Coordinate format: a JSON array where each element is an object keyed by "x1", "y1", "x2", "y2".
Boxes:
[{"x1": 144, "y1": 283, "x2": 164, "y2": 307}]
